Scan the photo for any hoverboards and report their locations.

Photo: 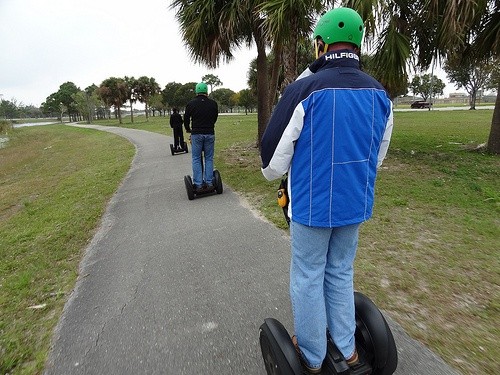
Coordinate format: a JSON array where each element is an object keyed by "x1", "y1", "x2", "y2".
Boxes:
[
  {"x1": 170, "y1": 121, "x2": 188, "y2": 155},
  {"x1": 184, "y1": 130, "x2": 223, "y2": 199},
  {"x1": 259, "y1": 174, "x2": 398, "y2": 375}
]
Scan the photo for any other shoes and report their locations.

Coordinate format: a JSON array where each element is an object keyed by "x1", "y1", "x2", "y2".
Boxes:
[
  {"x1": 345, "y1": 346, "x2": 359, "y2": 366},
  {"x1": 193, "y1": 183, "x2": 203, "y2": 190},
  {"x1": 204, "y1": 183, "x2": 214, "y2": 189},
  {"x1": 292, "y1": 334, "x2": 321, "y2": 373}
]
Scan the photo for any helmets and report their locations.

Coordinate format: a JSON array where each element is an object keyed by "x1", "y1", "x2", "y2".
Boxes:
[
  {"x1": 196, "y1": 83, "x2": 208, "y2": 96},
  {"x1": 313, "y1": 7, "x2": 364, "y2": 50}
]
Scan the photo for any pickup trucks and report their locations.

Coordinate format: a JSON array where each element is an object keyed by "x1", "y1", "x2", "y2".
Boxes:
[{"x1": 410, "y1": 101, "x2": 432, "y2": 108}]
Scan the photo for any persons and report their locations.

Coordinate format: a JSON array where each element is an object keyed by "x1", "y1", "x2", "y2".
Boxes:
[
  {"x1": 258, "y1": 8, "x2": 394, "y2": 374},
  {"x1": 183, "y1": 83, "x2": 218, "y2": 190},
  {"x1": 170, "y1": 107, "x2": 184, "y2": 150}
]
